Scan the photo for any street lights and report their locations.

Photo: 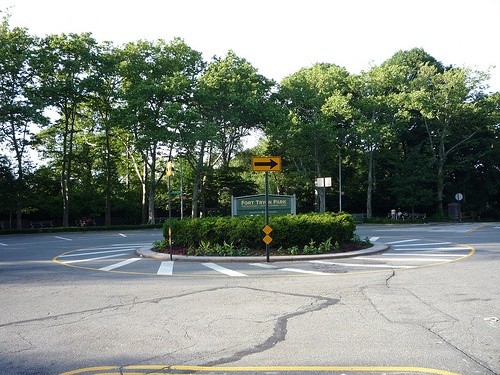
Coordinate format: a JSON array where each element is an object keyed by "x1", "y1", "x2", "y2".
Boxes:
[{"x1": 166, "y1": 161, "x2": 175, "y2": 261}]
